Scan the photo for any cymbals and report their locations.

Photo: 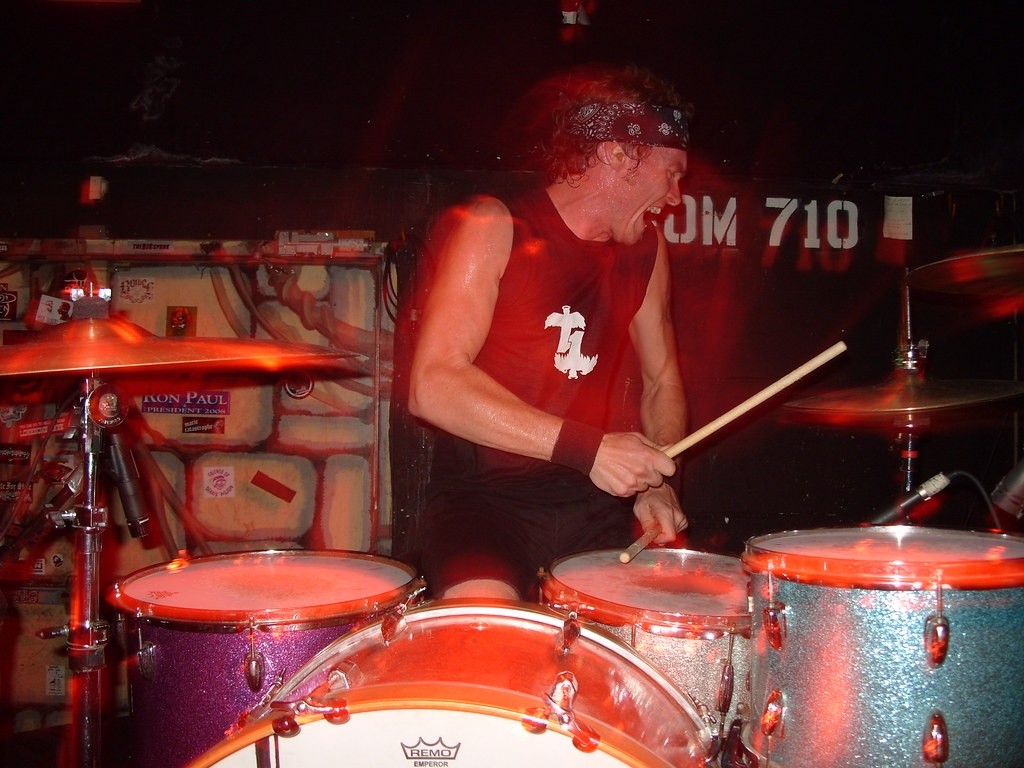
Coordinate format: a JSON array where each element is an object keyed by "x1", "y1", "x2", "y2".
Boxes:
[
  {"x1": 779, "y1": 377, "x2": 1024, "y2": 430},
  {"x1": 906, "y1": 241, "x2": 1024, "y2": 298},
  {"x1": 0, "y1": 317, "x2": 363, "y2": 377}
]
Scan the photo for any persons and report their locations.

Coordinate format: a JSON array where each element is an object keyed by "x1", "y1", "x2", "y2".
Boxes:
[{"x1": 401, "y1": 62, "x2": 697, "y2": 604}]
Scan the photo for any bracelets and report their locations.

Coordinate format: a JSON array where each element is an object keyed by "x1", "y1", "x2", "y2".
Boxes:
[{"x1": 552, "y1": 419, "x2": 604, "y2": 477}]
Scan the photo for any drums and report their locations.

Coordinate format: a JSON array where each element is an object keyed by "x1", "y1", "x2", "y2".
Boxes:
[
  {"x1": 738, "y1": 522, "x2": 1023, "y2": 768},
  {"x1": 534, "y1": 545, "x2": 752, "y2": 742},
  {"x1": 181, "y1": 596, "x2": 726, "y2": 768},
  {"x1": 100, "y1": 546, "x2": 429, "y2": 768}
]
